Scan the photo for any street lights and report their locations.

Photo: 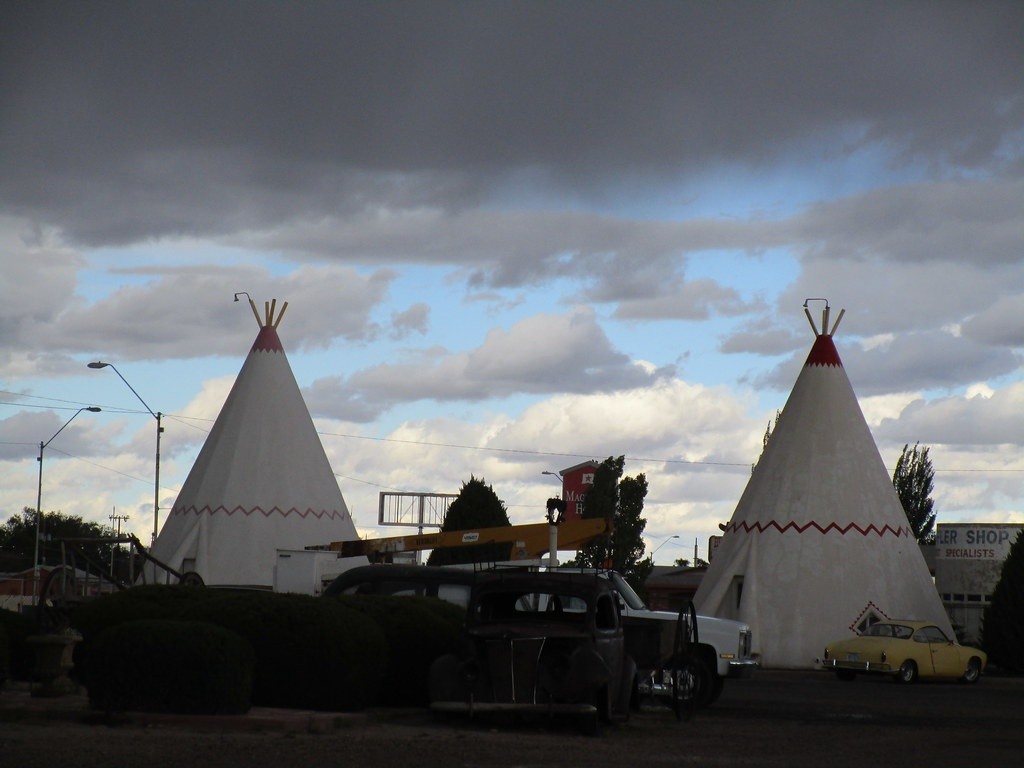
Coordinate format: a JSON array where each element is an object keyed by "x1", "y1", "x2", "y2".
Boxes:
[
  {"x1": 32, "y1": 407, "x2": 102, "y2": 607},
  {"x1": 87, "y1": 361, "x2": 164, "y2": 587}
]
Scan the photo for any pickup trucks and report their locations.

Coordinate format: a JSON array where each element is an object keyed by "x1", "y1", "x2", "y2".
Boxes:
[{"x1": 300, "y1": 516, "x2": 760, "y2": 713}]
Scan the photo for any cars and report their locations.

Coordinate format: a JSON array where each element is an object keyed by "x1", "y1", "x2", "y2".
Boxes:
[{"x1": 823, "y1": 619, "x2": 988, "y2": 685}]
